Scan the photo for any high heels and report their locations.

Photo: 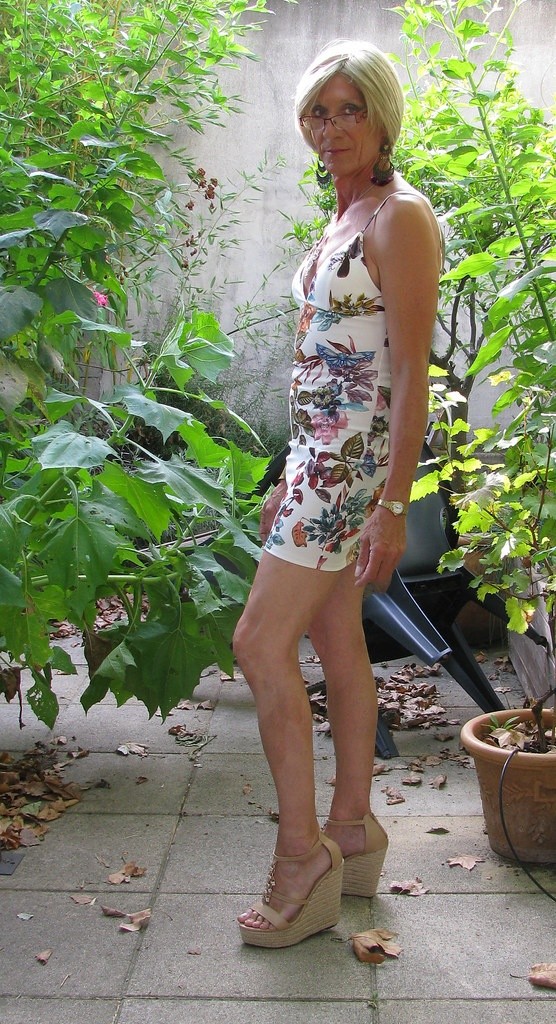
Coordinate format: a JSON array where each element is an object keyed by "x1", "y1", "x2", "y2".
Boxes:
[
  {"x1": 323, "y1": 813, "x2": 387, "y2": 897},
  {"x1": 239, "y1": 831, "x2": 346, "y2": 946}
]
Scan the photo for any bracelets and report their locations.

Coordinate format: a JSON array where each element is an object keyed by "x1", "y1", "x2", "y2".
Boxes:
[{"x1": 278, "y1": 465, "x2": 286, "y2": 481}]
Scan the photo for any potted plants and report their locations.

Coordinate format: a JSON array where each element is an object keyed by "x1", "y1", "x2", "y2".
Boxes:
[{"x1": 381, "y1": 0, "x2": 556, "y2": 871}]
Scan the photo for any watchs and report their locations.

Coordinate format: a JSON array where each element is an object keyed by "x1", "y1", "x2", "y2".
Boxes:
[{"x1": 378, "y1": 499, "x2": 407, "y2": 516}]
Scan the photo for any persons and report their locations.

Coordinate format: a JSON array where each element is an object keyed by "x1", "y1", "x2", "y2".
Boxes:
[{"x1": 233, "y1": 40, "x2": 443, "y2": 948}]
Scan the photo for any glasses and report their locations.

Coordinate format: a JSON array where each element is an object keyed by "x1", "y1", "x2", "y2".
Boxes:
[{"x1": 298, "y1": 106, "x2": 368, "y2": 132}]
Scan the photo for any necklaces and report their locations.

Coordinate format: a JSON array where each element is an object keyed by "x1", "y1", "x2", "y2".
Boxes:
[{"x1": 304, "y1": 182, "x2": 377, "y2": 279}]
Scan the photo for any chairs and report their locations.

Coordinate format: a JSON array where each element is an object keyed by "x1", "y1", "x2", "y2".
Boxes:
[{"x1": 244, "y1": 419, "x2": 548, "y2": 760}]
